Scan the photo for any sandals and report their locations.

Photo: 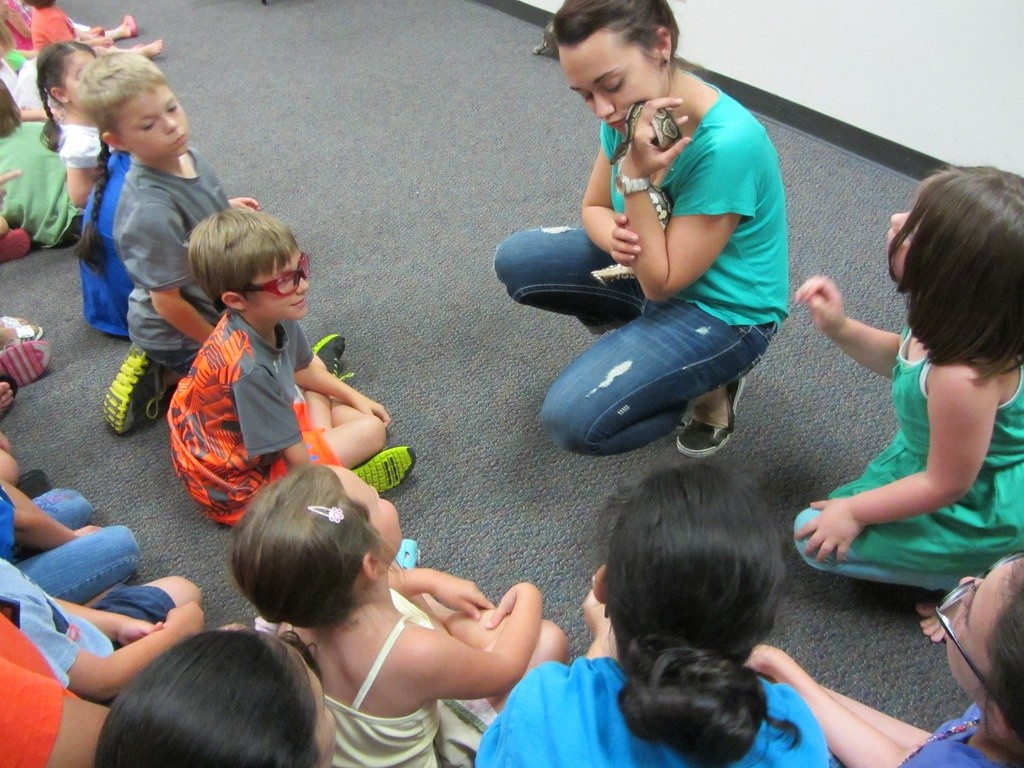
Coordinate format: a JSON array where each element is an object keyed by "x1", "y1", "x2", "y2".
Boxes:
[
  {"x1": 393, "y1": 539, "x2": 422, "y2": 572},
  {"x1": 0, "y1": 317, "x2": 52, "y2": 416}
]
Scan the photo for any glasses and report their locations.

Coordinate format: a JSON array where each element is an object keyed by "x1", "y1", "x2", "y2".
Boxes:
[
  {"x1": 233, "y1": 252, "x2": 309, "y2": 296},
  {"x1": 936, "y1": 551, "x2": 1024, "y2": 740}
]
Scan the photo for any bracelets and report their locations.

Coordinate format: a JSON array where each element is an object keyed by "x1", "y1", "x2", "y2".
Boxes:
[{"x1": 897, "y1": 718, "x2": 980, "y2": 768}]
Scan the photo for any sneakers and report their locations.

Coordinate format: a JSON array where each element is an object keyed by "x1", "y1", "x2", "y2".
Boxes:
[
  {"x1": 310, "y1": 335, "x2": 357, "y2": 383},
  {"x1": 349, "y1": 447, "x2": 416, "y2": 496},
  {"x1": 676, "y1": 377, "x2": 746, "y2": 458},
  {"x1": 105, "y1": 340, "x2": 166, "y2": 435}
]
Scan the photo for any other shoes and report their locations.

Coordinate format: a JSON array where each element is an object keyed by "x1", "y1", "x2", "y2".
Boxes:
[
  {"x1": 124, "y1": 14, "x2": 137, "y2": 38},
  {"x1": 0, "y1": 229, "x2": 31, "y2": 262}
]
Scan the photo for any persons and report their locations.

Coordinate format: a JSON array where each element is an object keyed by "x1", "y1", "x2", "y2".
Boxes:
[
  {"x1": 230, "y1": 463, "x2": 567, "y2": 768},
  {"x1": 473, "y1": 460, "x2": 830, "y2": 768},
  {"x1": 1, "y1": 0, "x2": 417, "y2": 767},
  {"x1": 743, "y1": 551, "x2": 1024, "y2": 768},
  {"x1": 793, "y1": 166, "x2": 1024, "y2": 597},
  {"x1": 493, "y1": 1, "x2": 790, "y2": 459}
]
